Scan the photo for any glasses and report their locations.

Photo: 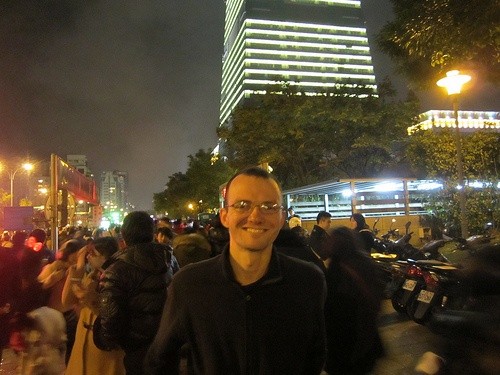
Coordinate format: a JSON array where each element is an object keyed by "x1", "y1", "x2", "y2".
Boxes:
[{"x1": 226, "y1": 198, "x2": 286, "y2": 216}]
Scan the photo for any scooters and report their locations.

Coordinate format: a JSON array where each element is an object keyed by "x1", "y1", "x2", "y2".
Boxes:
[{"x1": 370, "y1": 218, "x2": 500, "y2": 333}]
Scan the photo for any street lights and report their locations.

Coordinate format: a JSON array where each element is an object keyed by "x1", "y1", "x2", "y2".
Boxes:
[
  {"x1": 0, "y1": 164, "x2": 33, "y2": 207},
  {"x1": 436, "y1": 70, "x2": 471, "y2": 239}
]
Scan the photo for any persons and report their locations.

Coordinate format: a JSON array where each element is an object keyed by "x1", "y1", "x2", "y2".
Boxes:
[
  {"x1": 143, "y1": 167, "x2": 330, "y2": 375},
  {"x1": 0, "y1": 207, "x2": 387, "y2": 375}
]
